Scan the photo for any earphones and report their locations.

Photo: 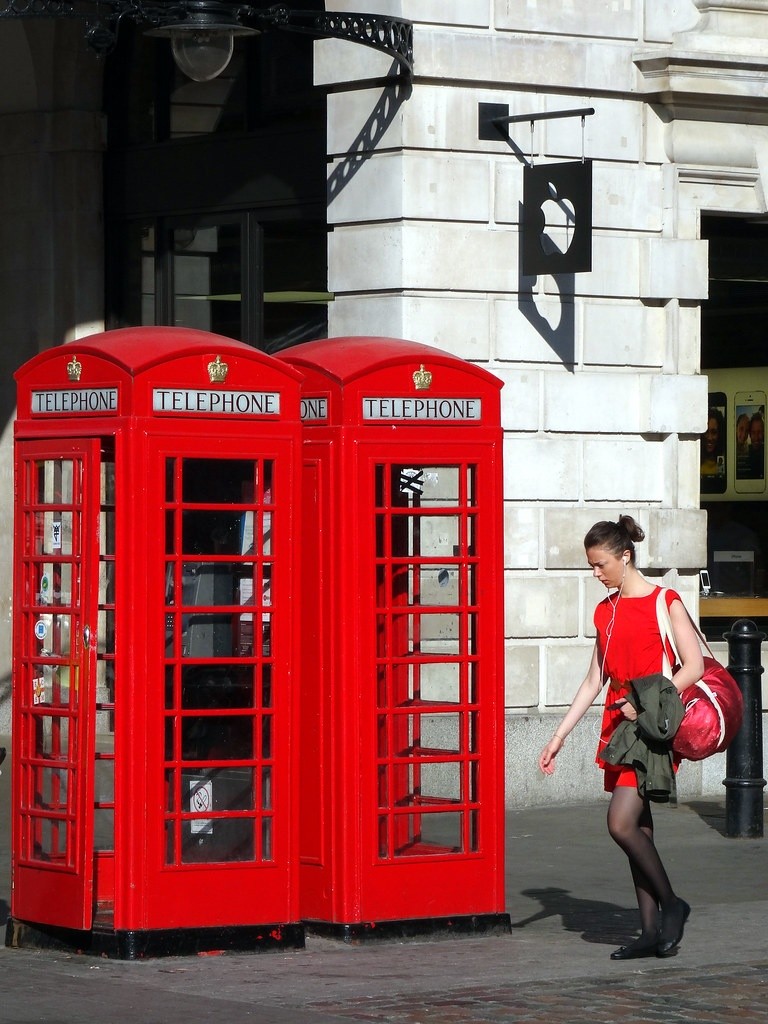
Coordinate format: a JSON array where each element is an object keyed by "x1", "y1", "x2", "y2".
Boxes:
[{"x1": 623, "y1": 556, "x2": 627, "y2": 562}]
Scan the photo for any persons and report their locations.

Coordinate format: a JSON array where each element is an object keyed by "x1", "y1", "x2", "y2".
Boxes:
[
  {"x1": 735, "y1": 405, "x2": 765, "y2": 479},
  {"x1": 538, "y1": 515, "x2": 705, "y2": 960},
  {"x1": 700, "y1": 408, "x2": 725, "y2": 480}
]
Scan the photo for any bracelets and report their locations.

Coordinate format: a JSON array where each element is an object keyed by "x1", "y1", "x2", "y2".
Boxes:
[{"x1": 553, "y1": 734, "x2": 564, "y2": 745}]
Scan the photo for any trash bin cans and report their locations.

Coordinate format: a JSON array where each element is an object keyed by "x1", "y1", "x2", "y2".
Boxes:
[{"x1": 167, "y1": 768, "x2": 266, "y2": 862}]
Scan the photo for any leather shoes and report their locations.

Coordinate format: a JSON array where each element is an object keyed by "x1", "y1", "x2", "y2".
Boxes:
[
  {"x1": 656, "y1": 897, "x2": 691, "y2": 956},
  {"x1": 611, "y1": 933, "x2": 662, "y2": 960}
]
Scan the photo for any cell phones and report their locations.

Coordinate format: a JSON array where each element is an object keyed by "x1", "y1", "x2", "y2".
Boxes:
[
  {"x1": 700, "y1": 392, "x2": 728, "y2": 494},
  {"x1": 733, "y1": 390, "x2": 767, "y2": 493},
  {"x1": 700, "y1": 570, "x2": 710, "y2": 588}
]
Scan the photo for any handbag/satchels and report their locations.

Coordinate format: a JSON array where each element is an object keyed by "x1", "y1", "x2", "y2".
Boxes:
[{"x1": 655, "y1": 588, "x2": 744, "y2": 762}]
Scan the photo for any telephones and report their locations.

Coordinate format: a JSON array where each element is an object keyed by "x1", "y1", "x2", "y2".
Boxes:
[{"x1": 164, "y1": 561, "x2": 215, "y2": 660}]
